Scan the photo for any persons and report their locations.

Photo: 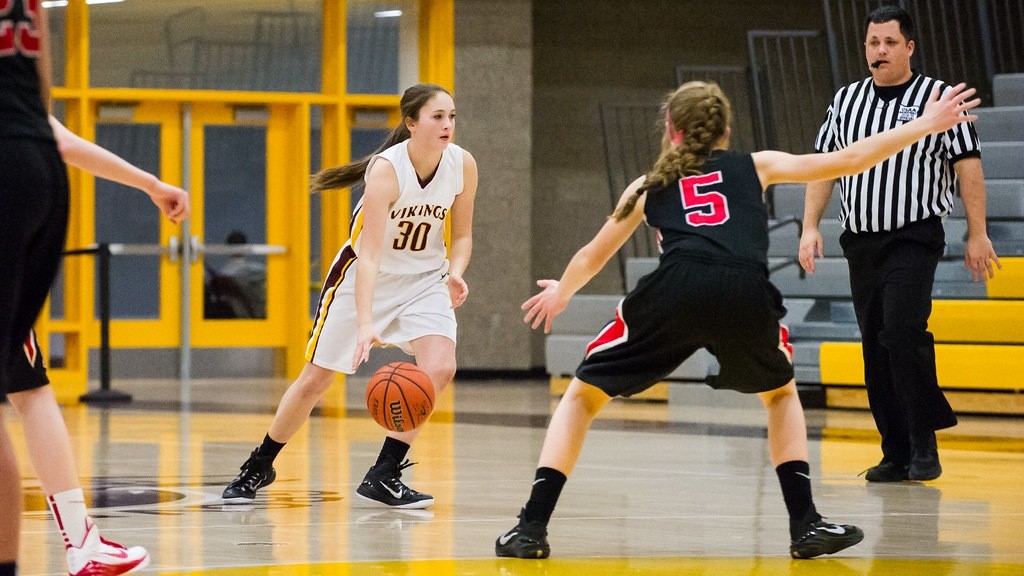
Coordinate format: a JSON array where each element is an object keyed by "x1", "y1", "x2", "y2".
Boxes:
[
  {"x1": 218, "y1": 231, "x2": 269, "y2": 315},
  {"x1": 799, "y1": 6, "x2": 1000, "y2": 481},
  {"x1": 495, "y1": 80, "x2": 983, "y2": 559},
  {"x1": 222, "y1": 84, "x2": 482, "y2": 507},
  {"x1": 0, "y1": 0, "x2": 192, "y2": 576}
]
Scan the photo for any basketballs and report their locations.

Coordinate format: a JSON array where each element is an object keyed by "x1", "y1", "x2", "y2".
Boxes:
[{"x1": 366, "y1": 361, "x2": 435, "y2": 432}]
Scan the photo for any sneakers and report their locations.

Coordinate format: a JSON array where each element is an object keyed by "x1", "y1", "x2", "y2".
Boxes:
[
  {"x1": 65, "y1": 516, "x2": 150, "y2": 576},
  {"x1": 789, "y1": 513, "x2": 864, "y2": 559},
  {"x1": 495, "y1": 506, "x2": 550, "y2": 559},
  {"x1": 908, "y1": 429, "x2": 942, "y2": 481},
  {"x1": 221, "y1": 446, "x2": 276, "y2": 504},
  {"x1": 865, "y1": 456, "x2": 909, "y2": 482},
  {"x1": 355, "y1": 458, "x2": 434, "y2": 508}
]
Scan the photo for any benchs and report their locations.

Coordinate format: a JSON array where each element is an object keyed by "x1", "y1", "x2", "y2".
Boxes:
[{"x1": 546, "y1": 73, "x2": 1022, "y2": 414}]
